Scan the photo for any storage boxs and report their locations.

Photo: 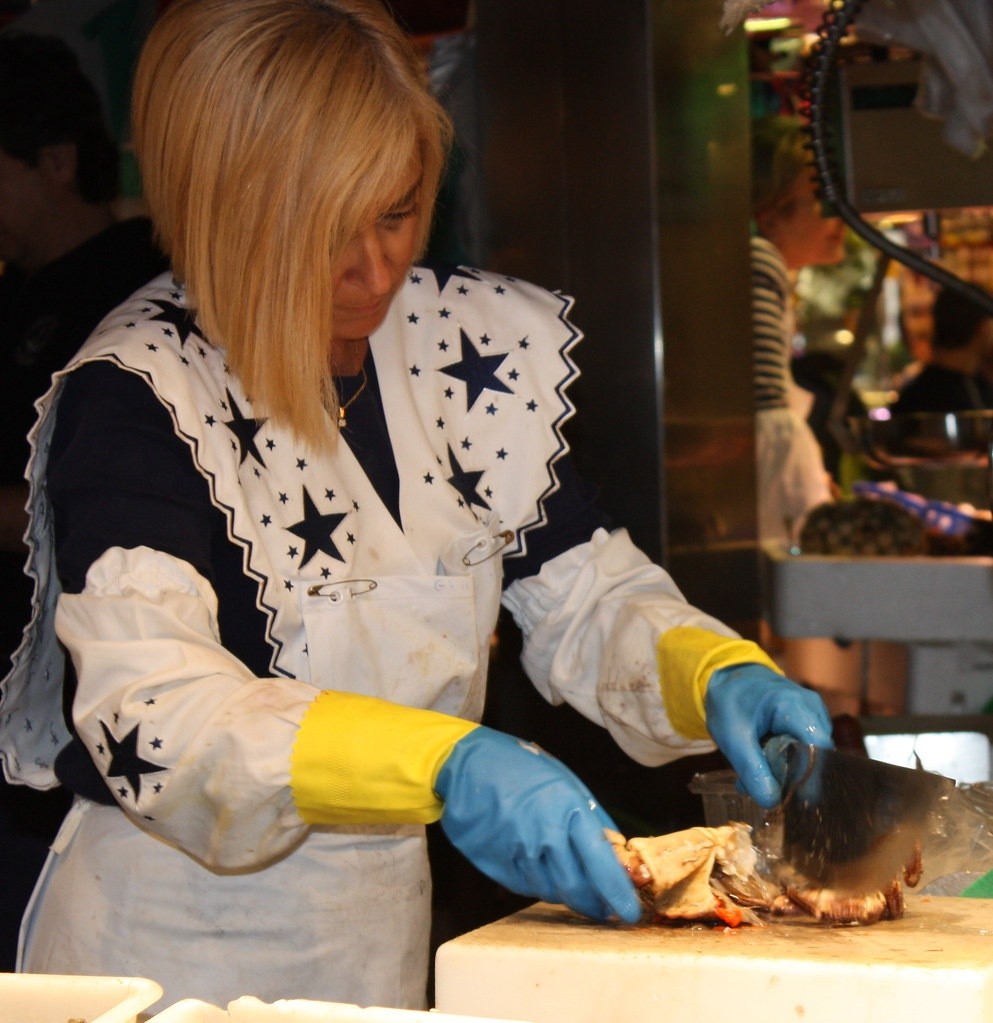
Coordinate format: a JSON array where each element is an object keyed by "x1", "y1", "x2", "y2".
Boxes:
[{"x1": 688, "y1": 768, "x2": 784, "y2": 854}]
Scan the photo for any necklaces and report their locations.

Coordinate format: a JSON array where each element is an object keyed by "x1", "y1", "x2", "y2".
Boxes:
[{"x1": 335, "y1": 351, "x2": 369, "y2": 427}]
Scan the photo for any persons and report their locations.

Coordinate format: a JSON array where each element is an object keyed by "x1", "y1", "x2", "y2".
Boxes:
[
  {"x1": 0, "y1": 0, "x2": 832, "y2": 1010},
  {"x1": 749, "y1": 102, "x2": 847, "y2": 547},
  {"x1": 893, "y1": 282, "x2": 993, "y2": 412}
]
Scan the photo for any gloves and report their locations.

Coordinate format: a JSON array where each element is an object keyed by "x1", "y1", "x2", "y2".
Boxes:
[
  {"x1": 289, "y1": 696, "x2": 644, "y2": 922},
  {"x1": 661, "y1": 622, "x2": 832, "y2": 809}
]
